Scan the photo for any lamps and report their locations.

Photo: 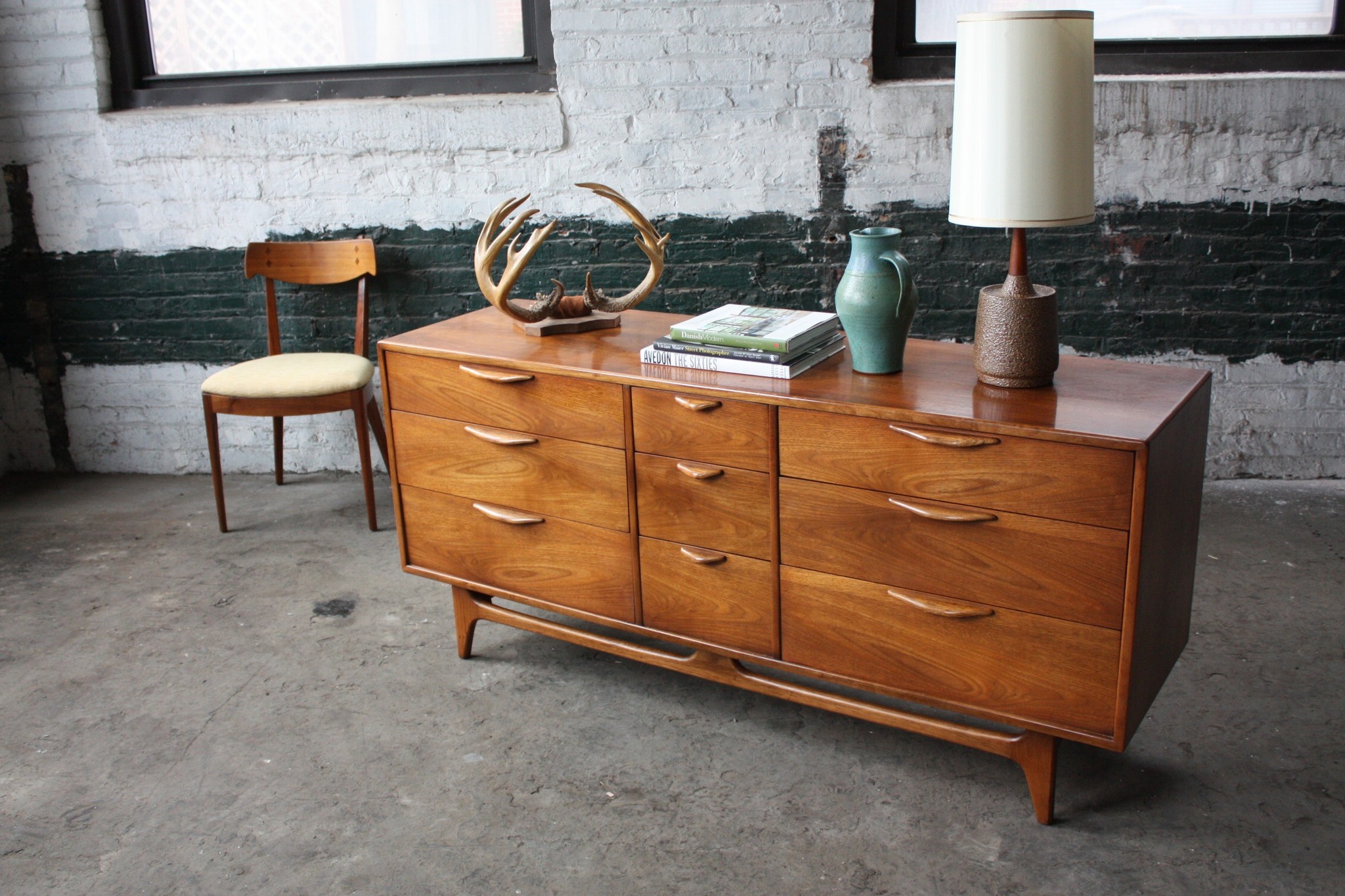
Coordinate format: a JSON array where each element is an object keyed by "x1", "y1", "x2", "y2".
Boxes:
[{"x1": 945, "y1": 10, "x2": 1096, "y2": 389}]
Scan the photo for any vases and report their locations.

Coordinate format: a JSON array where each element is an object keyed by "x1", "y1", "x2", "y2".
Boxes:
[{"x1": 835, "y1": 226, "x2": 921, "y2": 377}]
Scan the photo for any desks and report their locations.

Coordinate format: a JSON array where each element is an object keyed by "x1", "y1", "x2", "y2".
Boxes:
[{"x1": 376, "y1": 298, "x2": 1215, "y2": 828}]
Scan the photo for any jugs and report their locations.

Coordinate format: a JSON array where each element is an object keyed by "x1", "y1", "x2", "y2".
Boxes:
[{"x1": 834, "y1": 226, "x2": 919, "y2": 374}]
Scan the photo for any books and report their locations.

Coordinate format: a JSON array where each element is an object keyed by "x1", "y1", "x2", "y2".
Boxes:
[
  {"x1": 640, "y1": 335, "x2": 847, "y2": 380},
  {"x1": 671, "y1": 303, "x2": 840, "y2": 353},
  {"x1": 653, "y1": 324, "x2": 841, "y2": 365}
]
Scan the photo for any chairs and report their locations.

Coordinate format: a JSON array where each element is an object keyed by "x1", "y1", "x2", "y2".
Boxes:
[{"x1": 200, "y1": 238, "x2": 392, "y2": 533}]
